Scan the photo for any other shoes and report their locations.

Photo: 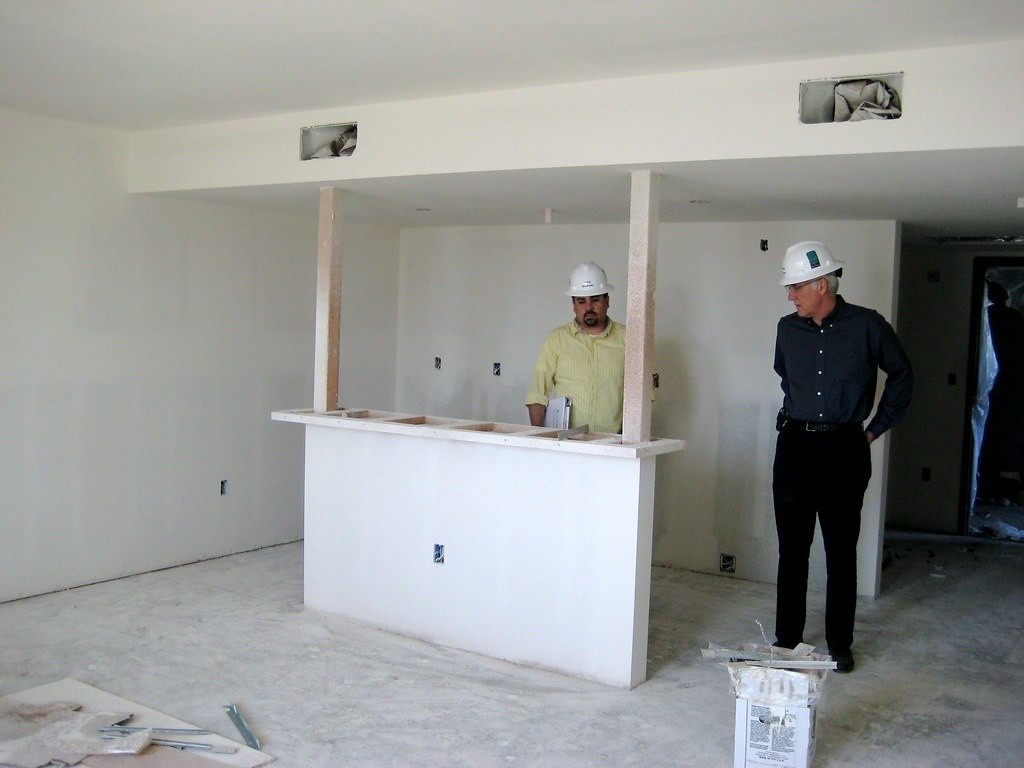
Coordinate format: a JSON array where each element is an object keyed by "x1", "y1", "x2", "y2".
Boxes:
[
  {"x1": 772, "y1": 641, "x2": 804, "y2": 651},
  {"x1": 830, "y1": 651, "x2": 855, "y2": 673}
]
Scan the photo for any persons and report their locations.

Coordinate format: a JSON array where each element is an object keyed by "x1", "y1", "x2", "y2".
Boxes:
[
  {"x1": 524, "y1": 261, "x2": 658, "y2": 435},
  {"x1": 771, "y1": 240, "x2": 916, "y2": 673}
]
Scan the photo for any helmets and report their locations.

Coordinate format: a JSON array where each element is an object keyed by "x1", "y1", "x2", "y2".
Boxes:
[
  {"x1": 780, "y1": 241, "x2": 846, "y2": 286},
  {"x1": 565, "y1": 261, "x2": 613, "y2": 298}
]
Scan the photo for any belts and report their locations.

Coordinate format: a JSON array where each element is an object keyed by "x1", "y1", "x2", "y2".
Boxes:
[{"x1": 788, "y1": 419, "x2": 864, "y2": 433}]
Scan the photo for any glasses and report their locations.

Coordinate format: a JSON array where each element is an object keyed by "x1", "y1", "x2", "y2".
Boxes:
[{"x1": 785, "y1": 277, "x2": 823, "y2": 292}]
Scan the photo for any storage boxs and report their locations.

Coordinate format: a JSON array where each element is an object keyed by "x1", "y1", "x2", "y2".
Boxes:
[{"x1": 728, "y1": 647, "x2": 831, "y2": 768}]
